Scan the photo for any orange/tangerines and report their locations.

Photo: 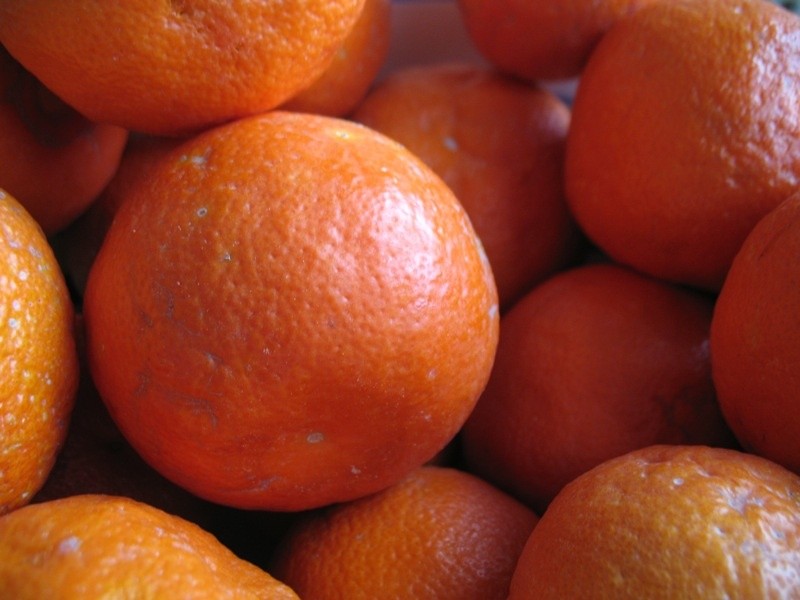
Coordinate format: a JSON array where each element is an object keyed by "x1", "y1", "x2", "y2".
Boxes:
[{"x1": 0, "y1": 0, "x2": 800, "y2": 600}]
